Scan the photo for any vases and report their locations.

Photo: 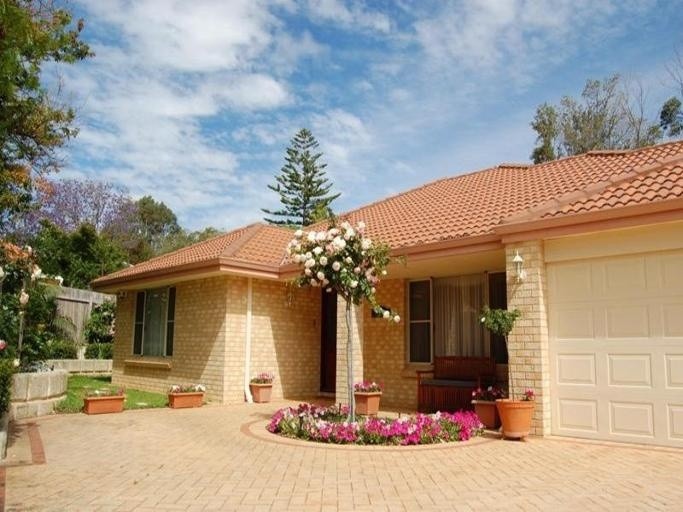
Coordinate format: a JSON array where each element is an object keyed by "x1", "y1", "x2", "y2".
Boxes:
[
  {"x1": 81, "y1": 395, "x2": 124, "y2": 414},
  {"x1": 351, "y1": 391, "x2": 382, "y2": 417},
  {"x1": 467, "y1": 399, "x2": 536, "y2": 442},
  {"x1": 163, "y1": 389, "x2": 203, "y2": 408},
  {"x1": 248, "y1": 384, "x2": 274, "y2": 403}
]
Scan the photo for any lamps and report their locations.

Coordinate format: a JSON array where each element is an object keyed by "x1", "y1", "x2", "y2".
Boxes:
[{"x1": 511, "y1": 249, "x2": 526, "y2": 285}]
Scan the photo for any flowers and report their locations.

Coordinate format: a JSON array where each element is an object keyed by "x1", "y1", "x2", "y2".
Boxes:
[
  {"x1": 250, "y1": 371, "x2": 278, "y2": 386},
  {"x1": 502, "y1": 387, "x2": 544, "y2": 402},
  {"x1": 76, "y1": 382, "x2": 126, "y2": 403},
  {"x1": 470, "y1": 385, "x2": 507, "y2": 402},
  {"x1": 167, "y1": 383, "x2": 205, "y2": 395},
  {"x1": 354, "y1": 379, "x2": 384, "y2": 395}
]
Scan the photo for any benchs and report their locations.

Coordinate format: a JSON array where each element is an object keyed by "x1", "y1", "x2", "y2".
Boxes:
[{"x1": 417, "y1": 355, "x2": 496, "y2": 415}]
